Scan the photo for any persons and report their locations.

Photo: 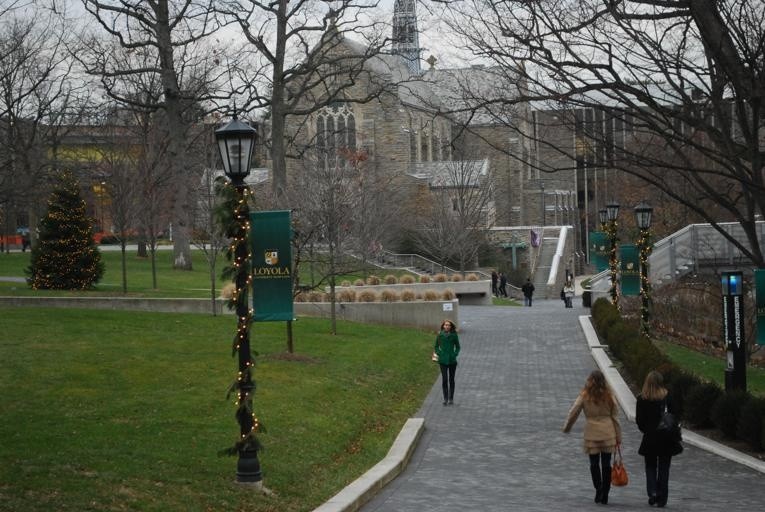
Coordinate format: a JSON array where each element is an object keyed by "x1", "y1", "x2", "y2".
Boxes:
[
  {"x1": 560, "y1": 283, "x2": 567, "y2": 307},
  {"x1": 492, "y1": 271, "x2": 498, "y2": 296},
  {"x1": 522, "y1": 279, "x2": 535, "y2": 306},
  {"x1": 21, "y1": 231, "x2": 32, "y2": 252},
  {"x1": 564, "y1": 371, "x2": 622, "y2": 505},
  {"x1": 435, "y1": 320, "x2": 461, "y2": 405},
  {"x1": 499, "y1": 272, "x2": 507, "y2": 298},
  {"x1": 636, "y1": 371, "x2": 683, "y2": 507},
  {"x1": 564, "y1": 283, "x2": 574, "y2": 308}
]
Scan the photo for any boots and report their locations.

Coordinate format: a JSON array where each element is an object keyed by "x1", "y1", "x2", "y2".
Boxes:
[
  {"x1": 602, "y1": 466, "x2": 611, "y2": 504},
  {"x1": 593, "y1": 476, "x2": 603, "y2": 503}
]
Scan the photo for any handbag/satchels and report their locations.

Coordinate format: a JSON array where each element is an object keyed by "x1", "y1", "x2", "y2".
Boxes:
[
  {"x1": 432, "y1": 352, "x2": 440, "y2": 362},
  {"x1": 657, "y1": 413, "x2": 683, "y2": 455},
  {"x1": 612, "y1": 461, "x2": 628, "y2": 485}
]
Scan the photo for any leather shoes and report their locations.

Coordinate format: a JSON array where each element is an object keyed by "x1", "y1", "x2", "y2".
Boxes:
[
  {"x1": 648, "y1": 494, "x2": 667, "y2": 507},
  {"x1": 443, "y1": 398, "x2": 454, "y2": 404}
]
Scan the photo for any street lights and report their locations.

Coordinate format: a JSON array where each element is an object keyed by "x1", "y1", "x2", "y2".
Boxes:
[
  {"x1": 598, "y1": 202, "x2": 653, "y2": 336},
  {"x1": 214, "y1": 99, "x2": 262, "y2": 483}
]
